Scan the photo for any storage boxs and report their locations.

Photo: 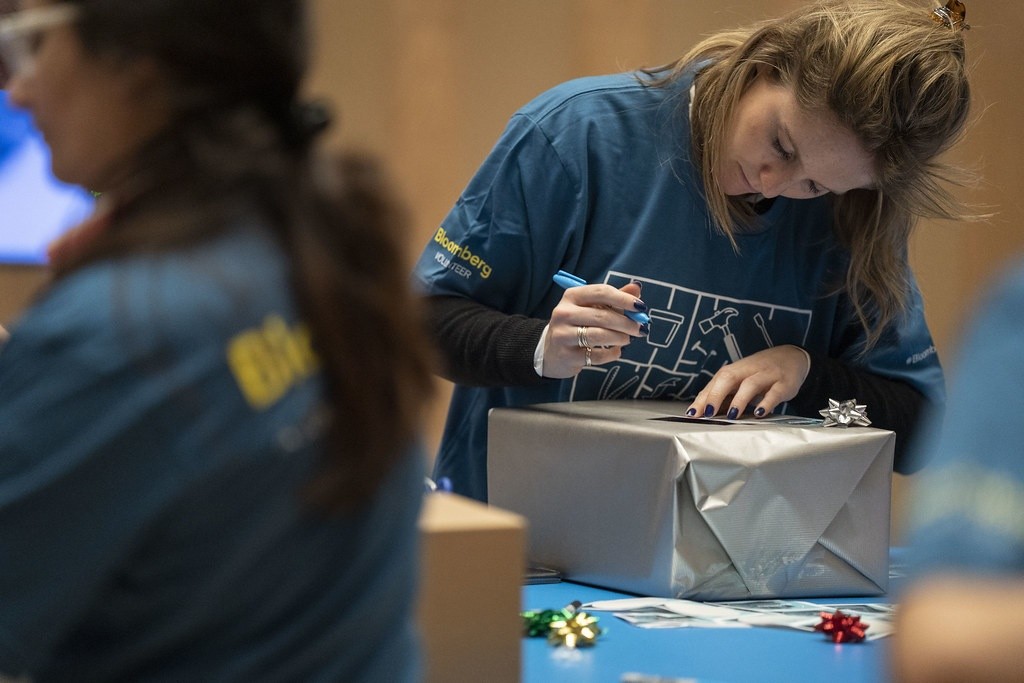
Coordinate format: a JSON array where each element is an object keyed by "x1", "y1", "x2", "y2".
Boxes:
[
  {"x1": 418, "y1": 492, "x2": 527, "y2": 683},
  {"x1": 486, "y1": 400, "x2": 896, "y2": 601}
]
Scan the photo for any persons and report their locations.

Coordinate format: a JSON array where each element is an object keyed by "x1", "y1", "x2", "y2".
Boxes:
[
  {"x1": 408, "y1": 1, "x2": 973, "y2": 507},
  {"x1": 0, "y1": 1, "x2": 437, "y2": 683}
]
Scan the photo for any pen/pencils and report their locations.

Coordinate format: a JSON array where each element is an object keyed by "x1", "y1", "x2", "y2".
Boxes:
[{"x1": 552, "y1": 269, "x2": 654, "y2": 325}]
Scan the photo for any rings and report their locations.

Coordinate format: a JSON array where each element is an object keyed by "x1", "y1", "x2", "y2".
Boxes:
[
  {"x1": 577, "y1": 326, "x2": 591, "y2": 348},
  {"x1": 586, "y1": 347, "x2": 592, "y2": 367}
]
